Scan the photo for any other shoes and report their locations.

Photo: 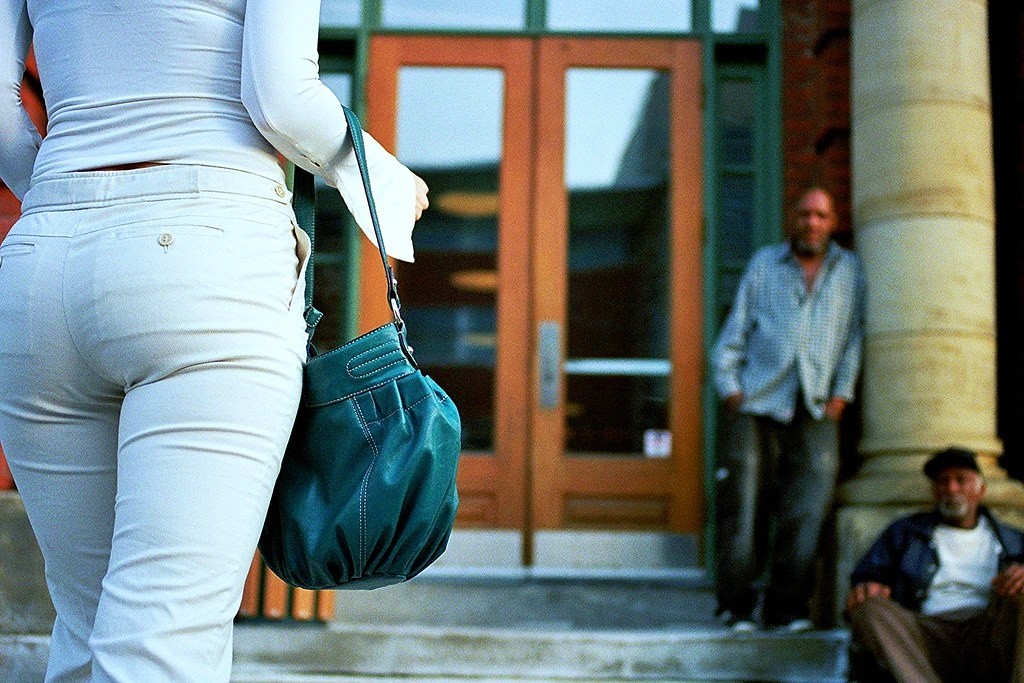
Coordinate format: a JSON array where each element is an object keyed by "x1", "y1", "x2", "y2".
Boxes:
[
  {"x1": 726, "y1": 616, "x2": 758, "y2": 635},
  {"x1": 781, "y1": 620, "x2": 813, "y2": 635}
]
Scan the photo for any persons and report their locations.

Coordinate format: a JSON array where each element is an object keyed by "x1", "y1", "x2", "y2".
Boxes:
[
  {"x1": 0, "y1": 0, "x2": 428, "y2": 683},
  {"x1": 710, "y1": 187, "x2": 863, "y2": 635},
  {"x1": 845, "y1": 448, "x2": 1024, "y2": 683}
]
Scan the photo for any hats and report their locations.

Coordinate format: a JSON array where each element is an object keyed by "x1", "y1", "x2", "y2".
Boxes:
[{"x1": 924, "y1": 448, "x2": 981, "y2": 481}]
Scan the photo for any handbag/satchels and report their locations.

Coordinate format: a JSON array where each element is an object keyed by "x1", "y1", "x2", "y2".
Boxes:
[{"x1": 259, "y1": 106, "x2": 461, "y2": 591}]
